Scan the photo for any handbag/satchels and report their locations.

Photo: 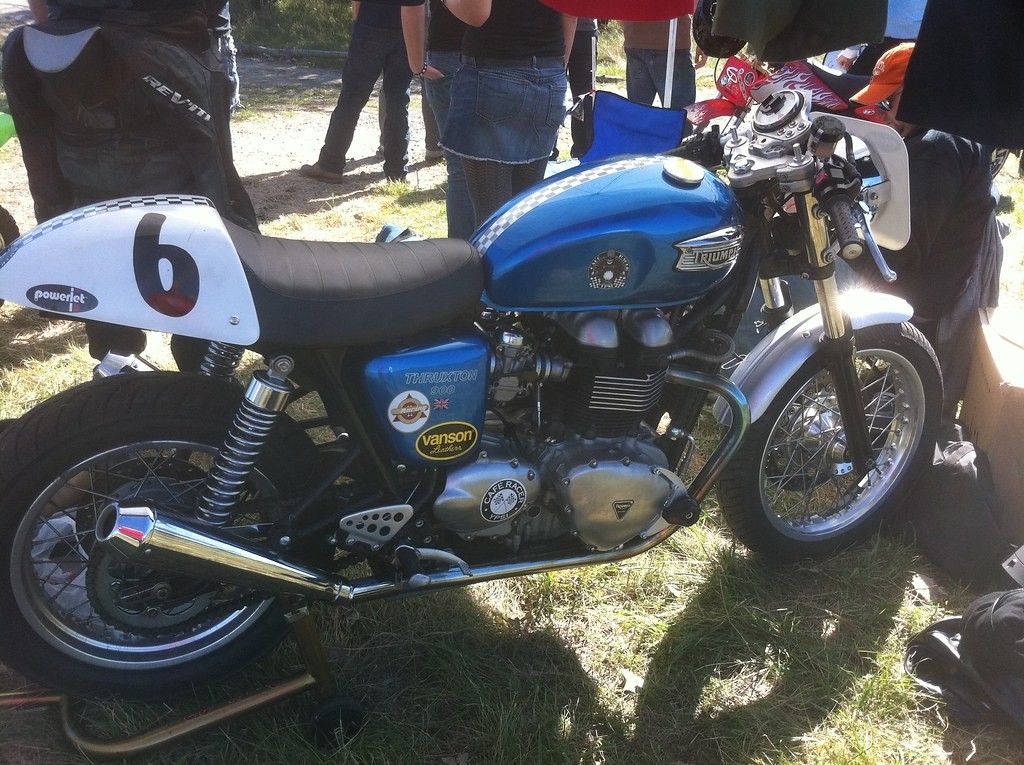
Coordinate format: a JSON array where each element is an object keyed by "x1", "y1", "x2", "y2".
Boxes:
[
  {"x1": 889, "y1": 421, "x2": 1016, "y2": 592},
  {"x1": 716, "y1": 54, "x2": 769, "y2": 108}
]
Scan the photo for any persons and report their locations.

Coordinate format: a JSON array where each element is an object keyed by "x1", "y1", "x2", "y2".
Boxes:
[
  {"x1": 618, "y1": 14, "x2": 709, "y2": 111},
  {"x1": 828, "y1": 0, "x2": 993, "y2": 462},
  {"x1": 300, "y1": 0, "x2": 598, "y2": 243},
  {"x1": 1, "y1": 0, "x2": 260, "y2": 382}
]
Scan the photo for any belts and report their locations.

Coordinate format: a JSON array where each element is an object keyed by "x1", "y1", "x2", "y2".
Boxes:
[{"x1": 459, "y1": 51, "x2": 565, "y2": 69}]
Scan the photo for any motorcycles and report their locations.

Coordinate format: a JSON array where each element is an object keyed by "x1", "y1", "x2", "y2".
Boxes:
[{"x1": 1, "y1": 0, "x2": 1024, "y2": 761}]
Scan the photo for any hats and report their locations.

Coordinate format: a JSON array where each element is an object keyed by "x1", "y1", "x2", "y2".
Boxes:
[{"x1": 848, "y1": 43, "x2": 917, "y2": 105}]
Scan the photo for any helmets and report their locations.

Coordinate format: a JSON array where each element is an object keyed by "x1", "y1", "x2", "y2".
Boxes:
[{"x1": 692, "y1": 1, "x2": 747, "y2": 58}]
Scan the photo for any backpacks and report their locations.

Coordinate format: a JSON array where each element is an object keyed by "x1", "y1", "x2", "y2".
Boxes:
[{"x1": 903, "y1": 587, "x2": 1024, "y2": 728}]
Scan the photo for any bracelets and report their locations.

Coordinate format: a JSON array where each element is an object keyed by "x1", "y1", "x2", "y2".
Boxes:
[{"x1": 415, "y1": 62, "x2": 427, "y2": 78}]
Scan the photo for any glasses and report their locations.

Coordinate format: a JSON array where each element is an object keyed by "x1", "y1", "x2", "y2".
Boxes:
[{"x1": 877, "y1": 90, "x2": 903, "y2": 111}]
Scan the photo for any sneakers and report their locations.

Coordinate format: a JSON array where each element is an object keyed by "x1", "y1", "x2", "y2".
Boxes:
[{"x1": 299, "y1": 161, "x2": 342, "y2": 184}]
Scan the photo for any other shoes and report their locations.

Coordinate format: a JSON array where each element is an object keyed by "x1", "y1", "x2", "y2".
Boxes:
[{"x1": 426, "y1": 147, "x2": 444, "y2": 158}]
